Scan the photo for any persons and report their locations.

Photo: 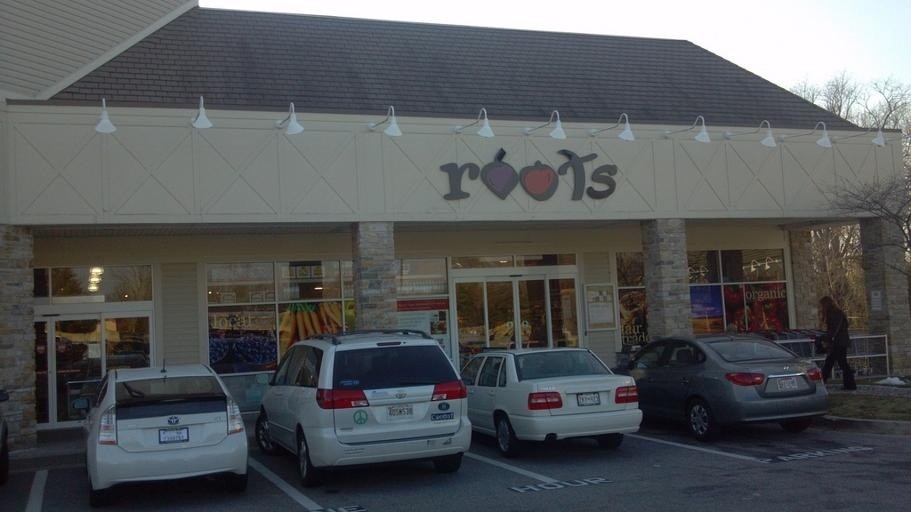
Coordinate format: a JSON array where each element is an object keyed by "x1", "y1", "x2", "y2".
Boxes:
[{"x1": 815, "y1": 296, "x2": 858, "y2": 390}]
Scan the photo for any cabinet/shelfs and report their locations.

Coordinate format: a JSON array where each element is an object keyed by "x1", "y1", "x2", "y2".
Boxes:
[
  {"x1": 63, "y1": 380, "x2": 104, "y2": 420},
  {"x1": 766, "y1": 331, "x2": 891, "y2": 385}
]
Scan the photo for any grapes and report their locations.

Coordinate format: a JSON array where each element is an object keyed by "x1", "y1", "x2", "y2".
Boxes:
[{"x1": 210, "y1": 334, "x2": 277, "y2": 364}]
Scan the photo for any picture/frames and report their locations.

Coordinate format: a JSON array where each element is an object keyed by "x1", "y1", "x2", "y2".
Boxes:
[{"x1": 583, "y1": 283, "x2": 618, "y2": 332}]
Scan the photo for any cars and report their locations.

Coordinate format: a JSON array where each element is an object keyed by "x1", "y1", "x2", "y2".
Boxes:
[
  {"x1": 78, "y1": 352, "x2": 147, "y2": 416},
  {"x1": 608, "y1": 329, "x2": 831, "y2": 444},
  {"x1": 456, "y1": 343, "x2": 646, "y2": 457},
  {"x1": 83, "y1": 360, "x2": 253, "y2": 508},
  {"x1": 1, "y1": 388, "x2": 11, "y2": 484}
]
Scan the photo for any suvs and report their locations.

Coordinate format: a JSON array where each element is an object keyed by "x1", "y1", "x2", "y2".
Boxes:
[{"x1": 253, "y1": 327, "x2": 476, "y2": 490}]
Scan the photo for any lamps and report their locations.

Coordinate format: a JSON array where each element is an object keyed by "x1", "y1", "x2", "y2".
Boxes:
[
  {"x1": 594, "y1": 112, "x2": 635, "y2": 141},
  {"x1": 281, "y1": 102, "x2": 304, "y2": 135},
  {"x1": 191, "y1": 97, "x2": 213, "y2": 130},
  {"x1": 372, "y1": 106, "x2": 400, "y2": 135},
  {"x1": 834, "y1": 124, "x2": 886, "y2": 147},
  {"x1": 87, "y1": 265, "x2": 104, "y2": 293},
  {"x1": 729, "y1": 122, "x2": 776, "y2": 147},
  {"x1": 688, "y1": 256, "x2": 783, "y2": 282},
  {"x1": 458, "y1": 107, "x2": 495, "y2": 137},
  {"x1": 528, "y1": 109, "x2": 567, "y2": 139},
  {"x1": 783, "y1": 122, "x2": 833, "y2": 147},
  {"x1": 94, "y1": 97, "x2": 118, "y2": 134},
  {"x1": 670, "y1": 114, "x2": 710, "y2": 142}
]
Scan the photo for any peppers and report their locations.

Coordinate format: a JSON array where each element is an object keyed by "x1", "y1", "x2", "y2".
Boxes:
[{"x1": 724, "y1": 283, "x2": 787, "y2": 334}]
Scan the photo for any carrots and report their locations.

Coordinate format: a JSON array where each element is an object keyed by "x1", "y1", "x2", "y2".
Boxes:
[{"x1": 279, "y1": 303, "x2": 342, "y2": 358}]
